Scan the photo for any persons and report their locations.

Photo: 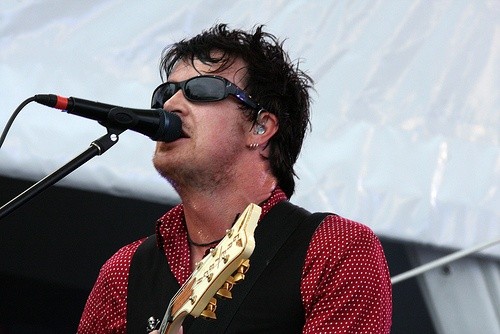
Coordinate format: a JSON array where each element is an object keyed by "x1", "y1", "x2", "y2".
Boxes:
[{"x1": 76, "y1": 19, "x2": 393, "y2": 334}]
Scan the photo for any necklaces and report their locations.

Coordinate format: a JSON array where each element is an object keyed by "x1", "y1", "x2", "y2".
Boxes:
[{"x1": 188, "y1": 196, "x2": 272, "y2": 248}]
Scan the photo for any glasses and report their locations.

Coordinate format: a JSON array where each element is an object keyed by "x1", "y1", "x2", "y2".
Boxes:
[{"x1": 151, "y1": 76, "x2": 262, "y2": 118}]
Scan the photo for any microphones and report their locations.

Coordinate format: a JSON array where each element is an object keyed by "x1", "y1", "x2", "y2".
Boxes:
[{"x1": 34, "y1": 93, "x2": 183, "y2": 143}]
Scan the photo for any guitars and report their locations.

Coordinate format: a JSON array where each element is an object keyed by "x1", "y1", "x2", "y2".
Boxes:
[{"x1": 141, "y1": 203, "x2": 264, "y2": 334}]
